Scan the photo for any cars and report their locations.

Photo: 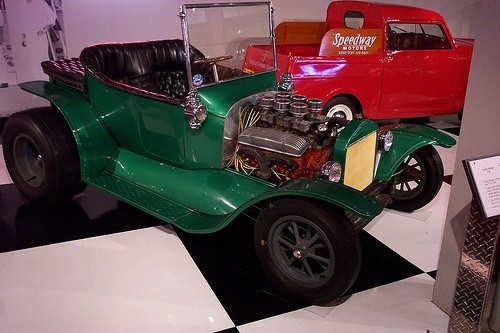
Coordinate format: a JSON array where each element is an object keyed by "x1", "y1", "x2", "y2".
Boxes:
[
  {"x1": 4, "y1": 2, "x2": 457, "y2": 303},
  {"x1": 241, "y1": 0, "x2": 478, "y2": 124}
]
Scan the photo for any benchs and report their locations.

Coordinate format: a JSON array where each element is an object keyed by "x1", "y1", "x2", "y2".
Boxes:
[{"x1": 80, "y1": 39, "x2": 213, "y2": 102}]
